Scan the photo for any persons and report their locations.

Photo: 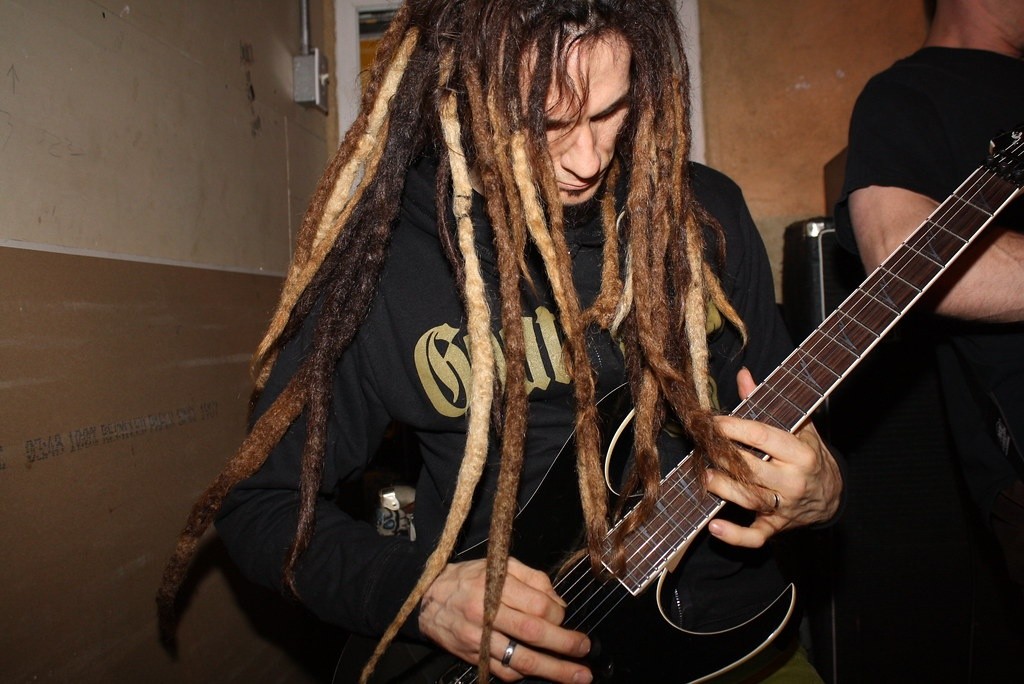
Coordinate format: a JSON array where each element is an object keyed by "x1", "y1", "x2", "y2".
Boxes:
[
  {"x1": 210, "y1": 0, "x2": 843, "y2": 684},
  {"x1": 848, "y1": 1, "x2": 1024, "y2": 581}
]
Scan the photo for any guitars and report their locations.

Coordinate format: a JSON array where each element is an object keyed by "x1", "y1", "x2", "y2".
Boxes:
[{"x1": 332, "y1": 123, "x2": 1024, "y2": 684}]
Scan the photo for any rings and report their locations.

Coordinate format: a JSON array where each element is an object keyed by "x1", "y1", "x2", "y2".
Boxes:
[
  {"x1": 501, "y1": 640, "x2": 517, "y2": 664},
  {"x1": 760, "y1": 492, "x2": 780, "y2": 515}
]
